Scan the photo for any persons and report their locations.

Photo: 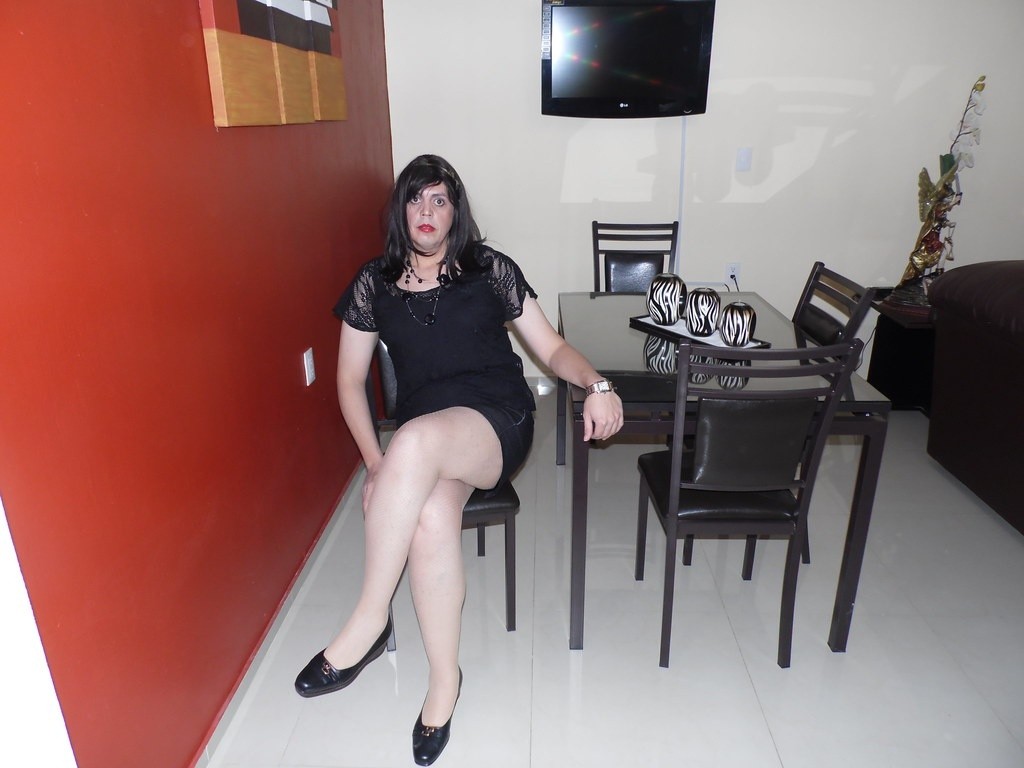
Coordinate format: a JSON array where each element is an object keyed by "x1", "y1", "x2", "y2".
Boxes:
[{"x1": 295, "y1": 152, "x2": 626, "y2": 766}]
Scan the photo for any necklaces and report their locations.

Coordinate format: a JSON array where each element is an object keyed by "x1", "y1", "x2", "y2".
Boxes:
[{"x1": 405, "y1": 257, "x2": 444, "y2": 327}]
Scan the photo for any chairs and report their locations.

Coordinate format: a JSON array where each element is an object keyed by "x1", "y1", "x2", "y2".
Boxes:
[
  {"x1": 377, "y1": 337, "x2": 520, "y2": 651},
  {"x1": 590, "y1": 221, "x2": 679, "y2": 447},
  {"x1": 667, "y1": 260, "x2": 874, "y2": 566},
  {"x1": 635, "y1": 337, "x2": 863, "y2": 669}
]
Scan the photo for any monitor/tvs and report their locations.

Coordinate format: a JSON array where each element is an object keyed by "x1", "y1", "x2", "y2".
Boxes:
[{"x1": 540, "y1": 0, "x2": 716, "y2": 119}]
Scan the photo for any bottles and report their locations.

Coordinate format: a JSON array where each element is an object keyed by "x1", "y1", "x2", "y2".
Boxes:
[
  {"x1": 686, "y1": 288, "x2": 721, "y2": 337},
  {"x1": 643, "y1": 334, "x2": 679, "y2": 374},
  {"x1": 688, "y1": 355, "x2": 718, "y2": 384},
  {"x1": 719, "y1": 302, "x2": 756, "y2": 347},
  {"x1": 716, "y1": 359, "x2": 751, "y2": 390},
  {"x1": 645, "y1": 273, "x2": 687, "y2": 325}
]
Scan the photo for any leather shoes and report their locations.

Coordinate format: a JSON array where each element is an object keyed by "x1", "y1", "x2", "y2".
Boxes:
[
  {"x1": 295, "y1": 615, "x2": 392, "y2": 698},
  {"x1": 411, "y1": 664, "x2": 463, "y2": 766}
]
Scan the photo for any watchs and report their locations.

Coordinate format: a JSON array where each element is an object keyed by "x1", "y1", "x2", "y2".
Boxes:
[{"x1": 585, "y1": 378, "x2": 614, "y2": 396}]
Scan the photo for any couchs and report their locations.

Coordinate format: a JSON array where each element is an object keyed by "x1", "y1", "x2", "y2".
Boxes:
[{"x1": 927, "y1": 260, "x2": 1024, "y2": 536}]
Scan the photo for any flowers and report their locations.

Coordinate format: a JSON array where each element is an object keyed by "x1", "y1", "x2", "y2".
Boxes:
[{"x1": 950, "y1": 75, "x2": 989, "y2": 197}]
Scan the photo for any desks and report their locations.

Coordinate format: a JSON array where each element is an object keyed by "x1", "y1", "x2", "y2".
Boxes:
[
  {"x1": 859, "y1": 287, "x2": 932, "y2": 418},
  {"x1": 558, "y1": 290, "x2": 889, "y2": 650}
]
